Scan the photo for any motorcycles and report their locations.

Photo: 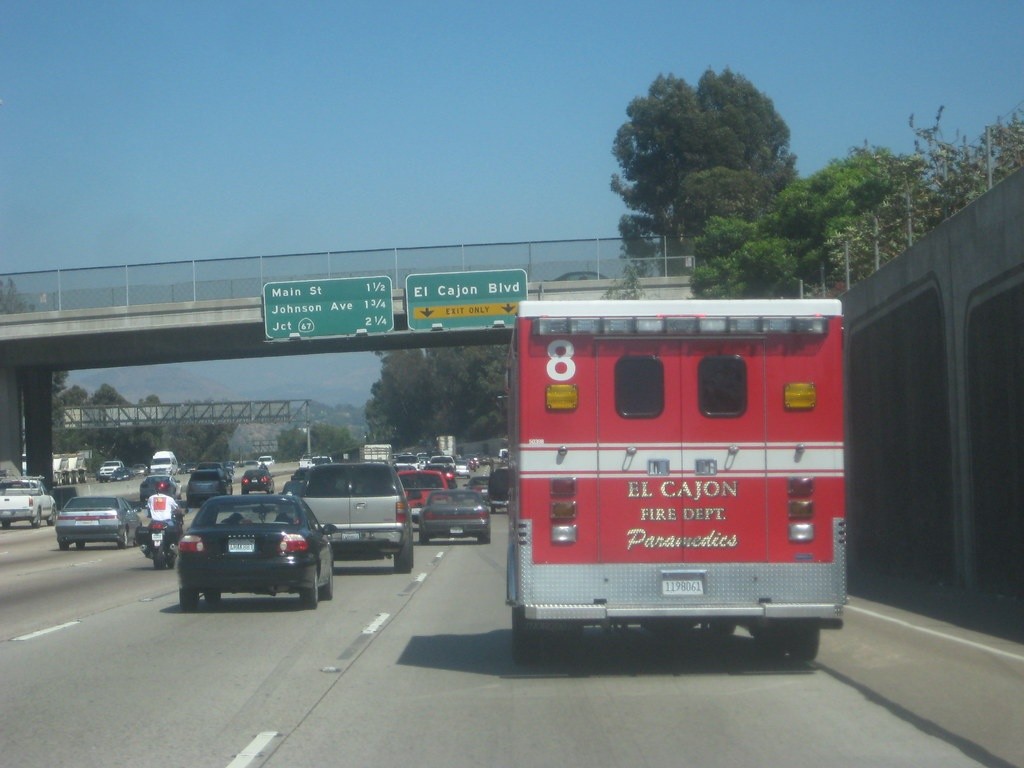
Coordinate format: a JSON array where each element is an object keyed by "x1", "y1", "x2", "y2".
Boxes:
[{"x1": 140, "y1": 496, "x2": 189, "y2": 570}]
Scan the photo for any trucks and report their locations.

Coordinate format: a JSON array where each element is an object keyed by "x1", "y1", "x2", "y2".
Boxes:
[{"x1": 488, "y1": 299, "x2": 849, "y2": 675}]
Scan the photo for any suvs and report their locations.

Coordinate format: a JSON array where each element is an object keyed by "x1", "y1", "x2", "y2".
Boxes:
[
  {"x1": 0, "y1": 476, "x2": 58, "y2": 528},
  {"x1": 99, "y1": 460, "x2": 125, "y2": 483},
  {"x1": 301, "y1": 459, "x2": 414, "y2": 573}
]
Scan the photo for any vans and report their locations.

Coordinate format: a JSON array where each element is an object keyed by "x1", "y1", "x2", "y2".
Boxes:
[{"x1": 150, "y1": 451, "x2": 178, "y2": 476}]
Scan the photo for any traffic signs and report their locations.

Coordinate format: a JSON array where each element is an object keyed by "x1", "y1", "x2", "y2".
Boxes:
[{"x1": 405, "y1": 269, "x2": 529, "y2": 329}]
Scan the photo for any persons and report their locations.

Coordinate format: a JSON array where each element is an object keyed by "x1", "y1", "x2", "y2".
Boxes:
[{"x1": 148, "y1": 481, "x2": 184, "y2": 543}]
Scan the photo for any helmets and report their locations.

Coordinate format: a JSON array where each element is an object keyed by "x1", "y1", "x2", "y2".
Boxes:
[{"x1": 155, "y1": 482, "x2": 169, "y2": 493}]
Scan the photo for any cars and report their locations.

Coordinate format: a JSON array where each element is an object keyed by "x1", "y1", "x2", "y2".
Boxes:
[
  {"x1": 545, "y1": 271, "x2": 608, "y2": 282},
  {"x1": 55, "y1": 496, "x2": 142, "y2": 550},
  {"x1": 140, "y1": 477, "x2": 182, "y2": 502},
  {"x1": 110, "y1": 467, "x2": 135, "y2": 482},
  {"x1": 131, "y1": 463, "x2": 149, "y2": 476},
  {"x1": 175, "y1": 494, "x2": 339, "y2": 611},
  {"x1": 278, "y1": 444, "x2": 510, "y2": 544},
  {"x1": 178, "y1": 455, "x2": 275, "y2": 508}
]
